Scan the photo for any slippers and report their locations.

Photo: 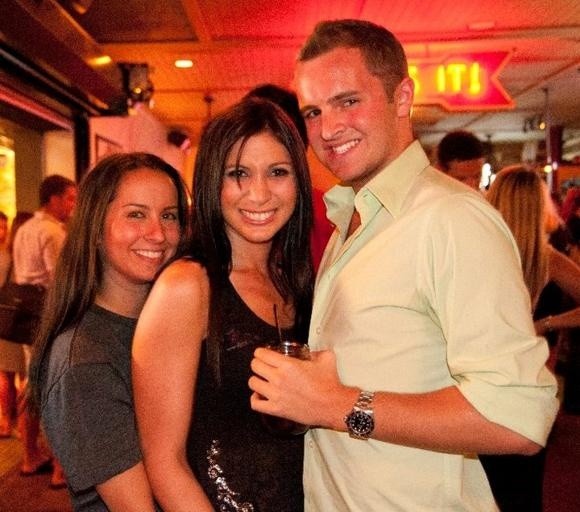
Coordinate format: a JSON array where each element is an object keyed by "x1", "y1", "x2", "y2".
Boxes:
[{"x1": 18, "y1": 453, "x2": 67, "y2": 489}]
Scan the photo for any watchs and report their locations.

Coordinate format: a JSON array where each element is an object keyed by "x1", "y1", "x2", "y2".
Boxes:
[
  {"x1": 343, "y1": 388, "x2": 376, "y2": 441},
  {"x1": 543, "y1": 314, "x2": 554, "y2": 331}
]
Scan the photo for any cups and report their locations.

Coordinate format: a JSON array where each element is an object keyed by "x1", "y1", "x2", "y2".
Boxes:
[{"x1": 260, "y1": 341, "x2": 309, "y2": 436}]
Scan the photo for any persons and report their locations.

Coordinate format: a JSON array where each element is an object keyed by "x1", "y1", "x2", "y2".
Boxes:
[
  {"x1": 248, "y1": 19, "x2": 562, "y2": 512},
  {"x1": 1, "y1": 170, "x2": 80, "y2": 490},
  {"x1": 438, "y1": 129, "x2": 486, "y2": 192},
  {"x1": 22, "y1": 153, "x2": 191, "y2": 510},
  {"x1": 479, "y1": 165, "x2": 579, "y2": 511},
  {"x1": 244, "y1": 82, "x2": 309, "y2": 149},
  {"x1": 132, "y1": 96, "x2": 318, "y2": 510},
  {"x1": 546, "y1": 179, "x2": 580, "y2": 416}
]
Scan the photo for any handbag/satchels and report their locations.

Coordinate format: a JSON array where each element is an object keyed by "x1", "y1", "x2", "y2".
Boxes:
[{"x1": 1, "y1": 280, "x2": 47, "y2": 348}]
